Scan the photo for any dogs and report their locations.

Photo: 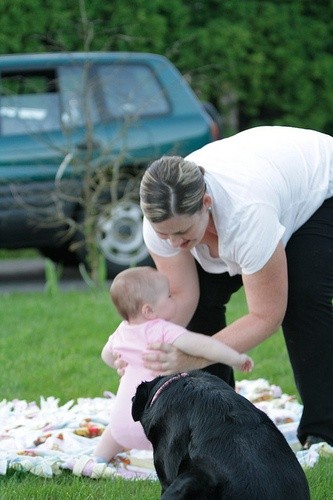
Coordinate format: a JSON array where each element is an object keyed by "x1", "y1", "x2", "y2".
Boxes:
[{"x1": 130, "y1": 363, "x2": 312, "y2": 500}]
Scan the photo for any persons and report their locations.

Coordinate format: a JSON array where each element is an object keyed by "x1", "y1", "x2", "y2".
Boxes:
[
  {"x1": 94, "y1": 266, "x2": 253, "y2": 462},
  {"x1": 140, "y1": 126, "x2": 333, "y2": 450}
]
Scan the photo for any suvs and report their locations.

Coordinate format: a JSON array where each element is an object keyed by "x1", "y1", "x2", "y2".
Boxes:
[{"x1": 0, "y1": 52, "x2": 223, "y2": 277}]
